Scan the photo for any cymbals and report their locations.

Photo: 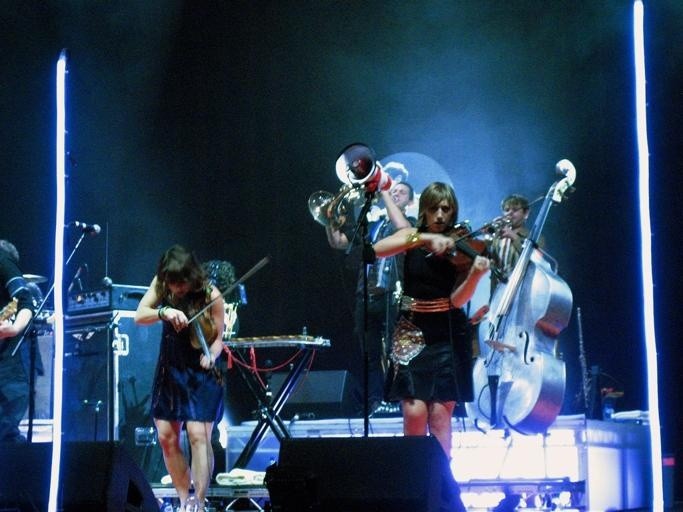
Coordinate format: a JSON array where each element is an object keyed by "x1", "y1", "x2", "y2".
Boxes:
[{"x1": 23, "y1": 275, "x2": 47, "y2": 285}]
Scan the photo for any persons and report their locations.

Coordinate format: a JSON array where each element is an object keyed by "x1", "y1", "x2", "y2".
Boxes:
[
  {"x1": 484, "y1": 194, "x2": 546, "y2": 439},
  {"x1": 371, "y1": 181, "x2": 489, "y2": 465},
  {"x1": 0, "y1": 239, "x2": 44, "y2": 512},
  {"x1": 325, "y1": 181, "x2": 417, "y2": 417},
  {"x1": 133, "y1": 246, "x2": 224, "y2": 512}
]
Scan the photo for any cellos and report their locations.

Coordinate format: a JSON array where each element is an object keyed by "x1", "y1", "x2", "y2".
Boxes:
[{"x1": 467, "y1": 159, "x2": 577, "y2": 432}]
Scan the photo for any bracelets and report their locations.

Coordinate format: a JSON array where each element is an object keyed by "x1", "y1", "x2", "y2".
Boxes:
[
  {"x1": 157, "y1": 305, "x2": 170, "y2": 320},
  {"x1": 406, "y1": 229, "x2": 419, "y2": 249}
]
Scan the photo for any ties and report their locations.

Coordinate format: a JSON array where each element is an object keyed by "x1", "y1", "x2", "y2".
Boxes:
[{"x1": 368, "y1": 217, "x2": 389, "y2": 287}]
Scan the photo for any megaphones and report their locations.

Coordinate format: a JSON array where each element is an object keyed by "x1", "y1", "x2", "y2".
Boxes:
[{"x1": 334, "y1": 142, "x2": 392, "y2": 191}]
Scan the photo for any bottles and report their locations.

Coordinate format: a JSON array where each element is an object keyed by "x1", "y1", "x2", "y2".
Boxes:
[{"x1": 160, "y1": 488, "x2": 200, "y2": 512}]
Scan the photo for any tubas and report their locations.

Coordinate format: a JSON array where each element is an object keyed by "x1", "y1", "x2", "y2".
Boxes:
[{"x1": 308, "y1": 163, "x2": 415, "y2": 227}]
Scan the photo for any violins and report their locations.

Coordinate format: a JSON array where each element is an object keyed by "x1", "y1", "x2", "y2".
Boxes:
[
  {"x1": 185, "y1": 287, "x2": 222, "y2": 385},
  {"x1": 437, "y1": 229, "x2": 510, "y2": 286}
]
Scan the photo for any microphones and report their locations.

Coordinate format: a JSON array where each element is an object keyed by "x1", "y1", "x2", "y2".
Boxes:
[{"x1": 68, "y1": 221, "x2": 102, "y2": 236}]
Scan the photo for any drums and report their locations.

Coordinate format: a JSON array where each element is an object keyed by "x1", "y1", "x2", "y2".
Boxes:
[{"x1": 32, "y1": 308, "x2": 53, "y2": 337}]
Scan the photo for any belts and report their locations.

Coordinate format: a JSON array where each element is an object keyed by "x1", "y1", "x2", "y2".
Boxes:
[{"x1": 358, "y1": 293, "x2": 385, "y2": 303}]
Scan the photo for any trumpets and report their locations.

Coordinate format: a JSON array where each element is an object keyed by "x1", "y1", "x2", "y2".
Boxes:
[{"x1": 483, "y1": 217, "x2": 512, "y2": 241}]
